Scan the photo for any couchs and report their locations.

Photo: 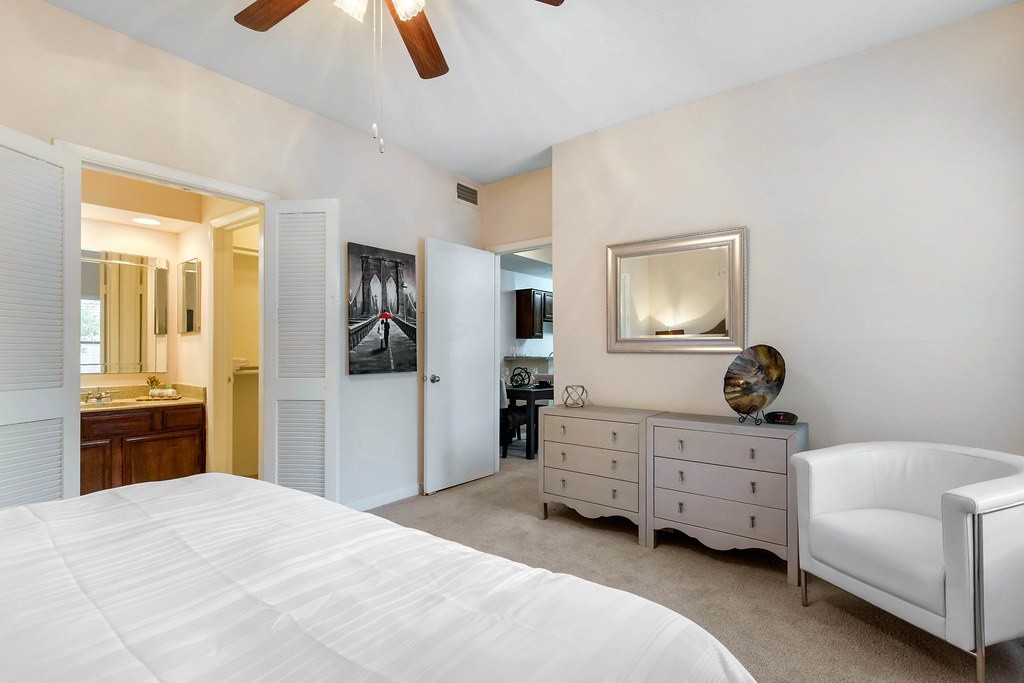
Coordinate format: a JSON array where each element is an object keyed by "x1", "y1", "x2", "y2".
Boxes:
[{"x1": 790, "y1": 441, "x2": 1024, "y2": 683}]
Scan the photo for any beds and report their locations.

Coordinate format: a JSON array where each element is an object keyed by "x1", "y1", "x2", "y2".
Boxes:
[{"x1": 0, "y1": 473, "x2": 757, "y2": 683}]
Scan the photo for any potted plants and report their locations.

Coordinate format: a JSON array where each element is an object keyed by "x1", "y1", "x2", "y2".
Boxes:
[{"x1": 145, "y1": 375, "x2": 160, "y2": 398}]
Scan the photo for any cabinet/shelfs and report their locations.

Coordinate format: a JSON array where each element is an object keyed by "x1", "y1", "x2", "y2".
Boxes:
[
  {"x1": 80, "y1": 404, "x2": 206, "y2": 496},
  {"x1": 515, "y1": 288, "x2": 543, "y2": 339},
  {"x1": 646, "y1": 412, "x2": 810, "y2": 586},
  {"x1": 537, "y1": 402, "x2": 669, "y2": 547},
  {"x1": 543, "y1": 290, "x2": 553, "y2": 322}
]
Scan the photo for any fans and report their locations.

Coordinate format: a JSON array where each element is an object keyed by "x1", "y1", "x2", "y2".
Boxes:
[{"x1": 233, "y1": 0, "x2": 565, "y2": 79}]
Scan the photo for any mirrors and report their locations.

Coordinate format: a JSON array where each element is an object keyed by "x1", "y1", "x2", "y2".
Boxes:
[
  {"x1": 605, "y1": 227, "x2": 749, "y2": 355},
  {"x1": 80, "y1": 249, "x2": 168, "y2": 374},
  {"x1": 176, "y1": 257, "x2": 201, "y2": 333}
]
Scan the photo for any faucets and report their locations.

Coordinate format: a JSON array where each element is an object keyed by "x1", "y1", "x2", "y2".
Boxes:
[{"x1": 95, "y1": 391, "x2": 102, "y2": 399}]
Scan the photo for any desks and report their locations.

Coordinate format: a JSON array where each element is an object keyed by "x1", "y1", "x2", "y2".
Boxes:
[{"x1": 506, "y1": 383, "x2": 555, "y2": 459}]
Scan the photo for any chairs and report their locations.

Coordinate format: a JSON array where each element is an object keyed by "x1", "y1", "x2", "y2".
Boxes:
[{"x1": 498, "y1": 378, "x2": 530, "y2": 459}]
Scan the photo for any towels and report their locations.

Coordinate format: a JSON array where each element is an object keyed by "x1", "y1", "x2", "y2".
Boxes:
[{"x1": 149, "y1": 389, "x2": 177, "y2": 397}]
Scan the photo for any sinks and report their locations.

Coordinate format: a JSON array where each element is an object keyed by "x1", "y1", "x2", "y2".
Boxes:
[{"x1": 80, "y1": 401, "x2": 145, "y2": 409}]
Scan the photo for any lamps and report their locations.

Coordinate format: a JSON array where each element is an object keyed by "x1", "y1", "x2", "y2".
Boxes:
[
  {"x1": 333, "y1": 0, "x2": 368, "y2": 23},
  {"x1": 392, "y1": 0, "x2": 426, "y2": 22}
]
